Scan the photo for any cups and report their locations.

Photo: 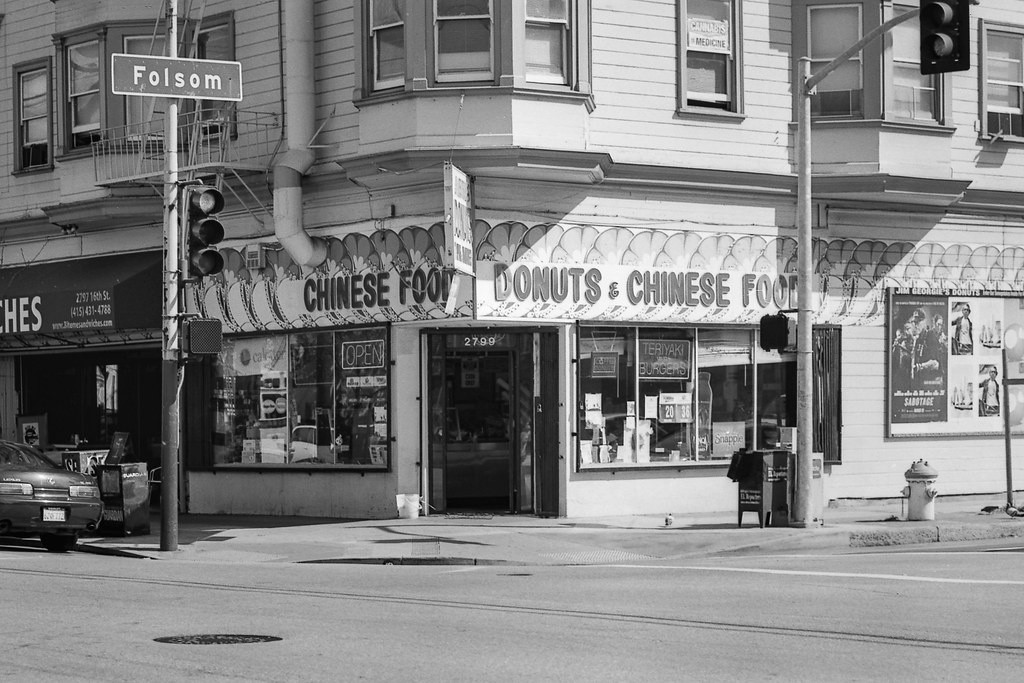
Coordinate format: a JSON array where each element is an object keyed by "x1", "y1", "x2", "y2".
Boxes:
[
  {"x1": 672, "y1": 451, "x2": 680, "y2": 462},
  {"x1": 599, "y1": 445, "x2": 611, "y2": 462}
]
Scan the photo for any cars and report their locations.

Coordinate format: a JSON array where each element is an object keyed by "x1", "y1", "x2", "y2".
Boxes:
[
  {"x1": 581, "y1": 414, "x2": 670, "y2": 459},
  {"x1": 0, "y1": 440, "x2": 104, "y2": 552},
  {"x1": 291, "y1": 425, "x2": 350, "y2": 463},
  {"x1": 650, "y1": 418, "x2": 783, "y2": 460}
]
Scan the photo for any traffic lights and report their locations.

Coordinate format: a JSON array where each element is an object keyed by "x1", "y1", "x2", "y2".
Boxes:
[
  {"x1": 759, "y1": 315, "x2": 797, "y2": 353},
  {"x1": 184, "y1": 185, "x2": 225, "y2": 283},
  {"x1": 183, "y1": 318, "x2": 222, "y2": 356},
  {"x1": 919, "y1": 0, "x2": 970, "y2": 76}
]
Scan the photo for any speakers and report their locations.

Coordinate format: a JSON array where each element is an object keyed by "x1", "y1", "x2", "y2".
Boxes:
[
  {"x1": 760, "y1": 314, "x2": 797, "y2": 350},
  {"x1": 190, "y1": 320, "x2": 223, "y2": 353}
]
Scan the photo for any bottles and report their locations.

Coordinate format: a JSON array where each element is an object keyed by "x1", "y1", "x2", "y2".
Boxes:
[{"x1": 694, "y1": 373, "x2": 712, "y2": 461}]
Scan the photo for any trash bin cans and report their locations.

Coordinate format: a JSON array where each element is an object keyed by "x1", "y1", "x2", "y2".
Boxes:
[
  {"x1": 61, "y1": 449, "x2": 111, "y2": 481},
  {"x1": 96, "y1": 430, "x2": 151, "y2": 536},
  {"x1": 727, "y1": 449, "x2": 824, "y2": 529}
]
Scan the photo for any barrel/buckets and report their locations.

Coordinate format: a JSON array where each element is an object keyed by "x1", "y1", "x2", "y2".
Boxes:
[{"x1": 397, "y1": 494, "x2": 422, "y2": 518}]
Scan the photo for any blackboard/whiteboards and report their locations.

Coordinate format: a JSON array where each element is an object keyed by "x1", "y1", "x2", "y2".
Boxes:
[{"x1": 638, "y1": 339, "x2": 690, "y2": 380}]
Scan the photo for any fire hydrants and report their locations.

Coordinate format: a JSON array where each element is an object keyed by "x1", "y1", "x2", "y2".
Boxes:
[{"x1": 902, "y1": 456, "x2": 938, "y2": 520}]
